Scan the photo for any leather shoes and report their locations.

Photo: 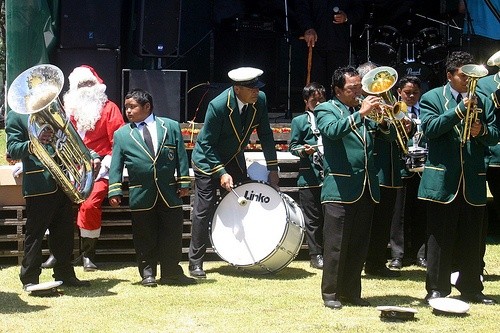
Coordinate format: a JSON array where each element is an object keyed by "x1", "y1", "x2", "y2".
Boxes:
[
  {"x1": 425, "y1": 290, "x2": 441, "y2": 304},
  {"x1": 160, "y1": 275, "x2": 197, "y2": 285},
  {"x1": 371, "y1": 265, "x2": 401, "y2": 277},
  {"x1": 323, "y1": 299, "x2": 341, "y2": 309},
  {"x1": 142, "y1": 276, "x2": 157, "y2": 287},
  {"x1": 189, "y1": 265, "x2": 207, "y2": 278},
  {"x1": 23, "y1": 282, "x2": 36, "y2": 292},
  {"x1": 55, "y1": 268, "x2": 90, "y2": 287},
  {"x1": 389, "y1": 258, "x2": 403, "y2": 268},
  {"x1": 460, "y1": 291, "x2": 494, "y2": 304},
  {"x1": 309, "y1": 254, "x2": 324, "y2": 268},
  {"x1": 336, "y1": 295, "x2": 371, "y2": 307},
  {"x1": 412, "y1": 257, "x2": 428, "y2": 268}
]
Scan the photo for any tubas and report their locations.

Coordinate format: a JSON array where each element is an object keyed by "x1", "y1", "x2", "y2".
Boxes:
[
  {"x1": 361, "y1": 66, "x2": 411, "y2": 155},
  {"x1": 7, "y1": 64, "x2": 94, "y2": 205}
]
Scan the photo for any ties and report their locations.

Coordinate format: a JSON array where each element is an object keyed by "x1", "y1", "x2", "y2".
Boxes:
[
  {"x1": 239, "y1": 105, "x2": 248, "y2": 136},
  {"x1": 456, "y1": 93, "x2": 461, "y2": 104},
  {"x1": 349, "y1": 106, "x2": 354, "y2": 114},
  {"x1": 140, "y1": 122, "x2": 156, "y2": 159},
  {"x1": 410, "y1": 106, "x2": 418, "y2": 134}
]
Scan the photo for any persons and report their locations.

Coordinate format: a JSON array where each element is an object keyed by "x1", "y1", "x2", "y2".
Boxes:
[
  {"x1": 107, "y1": 88, "x2": 197, "y2": 287},
  {"x1": 4, "y1": 102, "x2": 101, "y2": 294},
  {"x1": 459, "y1": 0, "x2": 500, "y2": 60},
  {"x1": 357, "y1": 51, "x2": 500, "y2": 277},
  {"x1": 41, "y1": 65, "x2": 125, "y2": 272},
  {"x1": 313, "y1": 66, "x2": 396, "y2": 309},
  {"x1": 417, "y1": 52, "x2": 498, "y2": 304},
  {"x1": 290, "y1": 84, "x2": 325, "y2": 269},
  {"x1": 287, "y1": 0, "x2": 364, "y2": 112},
  {"x1": 189, "y1": 68, "x2": 280, "y2": 278}
]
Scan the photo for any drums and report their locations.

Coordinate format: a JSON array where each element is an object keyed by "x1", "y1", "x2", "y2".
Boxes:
[
  {"x1": 209, "y1": 180, "x2": 305, "y2": 275},
  {"x1": 418, "y1": 28, "x2": 447, "y2": 64},
  {"x1": 370, "y1": 25, "x2": 399, "y2": 65},
  {"x1": 406, "y1": 118, "x2": 428, "y2": 158},
  {"x1": 395, "y1": 59, "x2": 435, "y2": 86}
]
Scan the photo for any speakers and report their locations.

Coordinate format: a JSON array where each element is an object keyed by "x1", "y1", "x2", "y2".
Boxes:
[
  {"x1": 131, "y1": 0, "x2": 181, "y2": 58},
  {"x1": 60, "y1": 0, "x2": 121, "y2": 49},
  {"x1": 56, "y1": 48, "x2": 121, "y2": 114}
]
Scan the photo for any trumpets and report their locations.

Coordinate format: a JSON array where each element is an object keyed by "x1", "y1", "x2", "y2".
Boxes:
[
  {"x1": 487, "y1": 50, "x2": 500, "y2": 69},
  {"x1": 355, "y1": 96, "x2": 408, "y2": 124},
  {"x1": 461, "y1": 64, "x2": 489, "y2": 143}
]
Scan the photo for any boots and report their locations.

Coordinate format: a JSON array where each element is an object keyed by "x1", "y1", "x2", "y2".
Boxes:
[
  {"x1": 41, "y1": 228, "x2": 55, "y2": 269},
  {"x1": 80, "y1": 227, "x2": 102, "y2": 271}
]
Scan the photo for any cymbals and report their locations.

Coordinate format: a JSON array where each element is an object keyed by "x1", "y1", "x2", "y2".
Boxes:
[{"x1": 435, "y1": 13, "x2": 465, "y2": 21}]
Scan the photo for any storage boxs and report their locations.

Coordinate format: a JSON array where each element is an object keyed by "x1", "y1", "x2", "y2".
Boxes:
[{"x1": 0, "y1": 166, "x2": 28, "y2": 207}]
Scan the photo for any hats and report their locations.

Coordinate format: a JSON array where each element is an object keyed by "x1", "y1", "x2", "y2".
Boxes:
[
  {"x1": 226, "y1": 67, "x2": 266, "y2": 90},
  {"x1": 68, "y1": 65, "x2": 104, "y2": 91},
  {"x1": 429, "y1": 297, "x2": 471, "y2": 317},
  {"x1": 451, "y1": 271, "x2": 484, "y2": 285},
  {"x1": 377, "y1": 305, "x2": 418, "y2": 322},
  {"x1": 26, "y1": 280, "x2": 64, "y2": 297}
]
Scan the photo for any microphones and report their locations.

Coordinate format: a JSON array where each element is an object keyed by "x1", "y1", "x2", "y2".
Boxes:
[{"x1": 334, "y1": 7, "x2": 340, "y2": 15}]
